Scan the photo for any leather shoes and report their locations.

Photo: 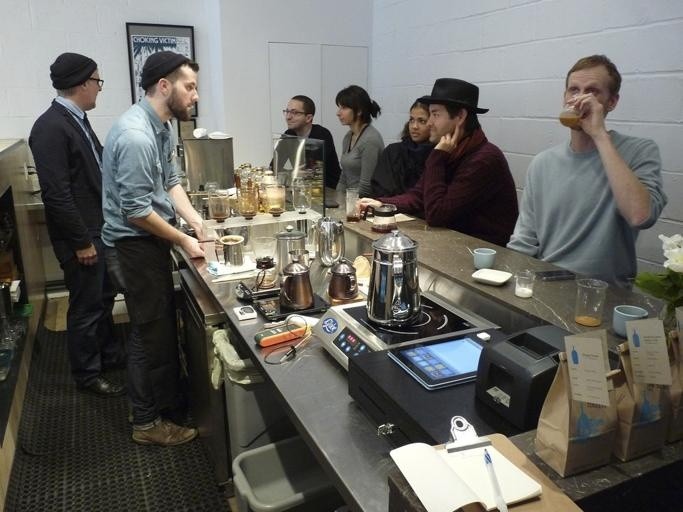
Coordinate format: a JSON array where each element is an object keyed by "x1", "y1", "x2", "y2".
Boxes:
[
  {"x1": 131, "y1": 416, "x2": 200, "y2": 446},
  {"x1": 73, "y1": 369, "x2": 128, "y2": 398},
  {"x1": 128, "y1": 403, "x2": 136, "y2": 425},
  {"x1": 100, "y1": 350, "x2": 129, "y2": 372}
]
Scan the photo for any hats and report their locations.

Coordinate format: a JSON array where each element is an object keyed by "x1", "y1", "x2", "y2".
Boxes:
[
  {"x1": 418, "y1": 77, "x2": 490, "y2": 115},
  {"x1": 140, "y1": 51, "x2": 190, "y2": 89},
  {"x1": 49, "y1": 52, "x2": 99, "y2": 91}
]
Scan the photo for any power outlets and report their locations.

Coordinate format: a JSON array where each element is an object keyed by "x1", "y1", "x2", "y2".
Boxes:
[{"x1": 23, "y1": 162, "x2": 29, "y2": 180}]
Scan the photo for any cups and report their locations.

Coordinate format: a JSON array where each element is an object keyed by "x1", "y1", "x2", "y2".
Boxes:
[
  {"x1": 515, "y1": 269, "x2": 536, "y2": 297},
  {"x1": 473, "y1": 249, "x2": 496, "y2": 270},
  {"x1": 237, "y1": 188, "x2": 258, "y2": 218},
  {"x1": 252, "y1": 236, "x2": 278, "y2": 259},
  {"x1": 209, "y1": 189, "x2": 230, "y2": 222},
  {"x1": 346, "y1": 188, "x2": 360, "y2": 198},
  {"x1": 345, "y1": 198, "x2": 360, "y2": 221},
  {"x1": 292, "y1": 184, "x2": 311, "y2": 213},
  {"x1": 613, "y1": 305, "x2": 648, "y2": 337},
  {"x1": 559, "y1": 101, "x2": 584, "y2": 127},
  {"x1": 574, "y1": 279, "x2": 606, "y2": 326},
  {"x1": 266, "y1": 185, "x2": 285, "y2": 216},
  {"x1": 220, "y1": 235, "x2": 244, "y2": 266}
]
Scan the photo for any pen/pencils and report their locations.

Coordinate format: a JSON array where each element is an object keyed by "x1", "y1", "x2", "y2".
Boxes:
[{"x1": 484, "y1": 448, "x2": 509, "y2": 512}]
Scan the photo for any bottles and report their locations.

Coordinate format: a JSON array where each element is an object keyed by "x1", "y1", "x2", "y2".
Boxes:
[{"x1": 255, "y1": 258, "x2": 278, "y2": 290}]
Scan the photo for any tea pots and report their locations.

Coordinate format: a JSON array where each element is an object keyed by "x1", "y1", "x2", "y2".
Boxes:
[
  {"x1": 276, "y1": 225, "x2": 307, "y2": 274},
  {"x1": 362, "y1": 204, "x2": 400, "y2": 233},
  {"x1": 366, "y1": 230, "x2": 420, "y2": 325},
  {"x1": 317, "y1": 216, "x2": 345, "y2": 266},
  {"x1": 279, "y1": 257, "x2": 313, "y2": 310}
]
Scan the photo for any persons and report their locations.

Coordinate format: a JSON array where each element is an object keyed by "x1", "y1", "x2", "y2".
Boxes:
[
  {"x1": 269, "y1": 95, "x2": 342, "y2": 189},
  {"x1": 506, "y1": 55, "x2": 668, "y2": 291},
  {"x1": 28, "y1": 53, "x2": 128, "y2": 399},
  {"x1": 358, "y1": 79, "x2": 519, "y2": 248},
  {"x1": 335, "y1": 85, "x2": 385, "y2": 198},
  {"x1": 370, "y1": 96, "x2": 437, "y2": 220},
  {"x1": 100, "y1": 51, "x2": 220, "y2": 447}
]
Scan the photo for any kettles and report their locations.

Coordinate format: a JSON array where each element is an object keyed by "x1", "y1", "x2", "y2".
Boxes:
[{"x1": 328, "y1": 259, "x2": 357, "y2": 300}]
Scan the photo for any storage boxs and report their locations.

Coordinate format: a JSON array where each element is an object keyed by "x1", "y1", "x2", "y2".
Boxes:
[{"x1": 232, "y1": 436, "x2": 334, "y2": 512}]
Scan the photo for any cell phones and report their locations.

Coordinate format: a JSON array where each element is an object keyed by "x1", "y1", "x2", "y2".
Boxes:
[{"x1": 535, "y1": 270, "x2": 575, "y2": 282}]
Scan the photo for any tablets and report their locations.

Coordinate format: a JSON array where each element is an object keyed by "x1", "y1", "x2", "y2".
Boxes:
[{"x1": 387, "y1": 332, "x2": 488, "y2": 392}]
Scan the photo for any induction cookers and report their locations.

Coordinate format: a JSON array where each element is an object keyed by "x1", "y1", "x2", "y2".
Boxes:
[
  {"x1": 256, "y1": 290, "x2": 329, "y2": 320},
  {"x1": 311, "y1": 290, "x2": 501, "y2": 374}
]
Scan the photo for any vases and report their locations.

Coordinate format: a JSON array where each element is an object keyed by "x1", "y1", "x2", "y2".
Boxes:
[{"x1": 657, "y1": 303, "x2": 677, "y2": 350}]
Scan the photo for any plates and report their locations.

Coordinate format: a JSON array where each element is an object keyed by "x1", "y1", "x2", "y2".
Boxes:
[{"x1": 472, "y1": 268, "x2": 513, "y2": 285}]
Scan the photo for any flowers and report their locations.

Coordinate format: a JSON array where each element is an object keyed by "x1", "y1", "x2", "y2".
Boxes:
[{"x1": 629, "y1": 233, "x2": 683, "y2": 347}]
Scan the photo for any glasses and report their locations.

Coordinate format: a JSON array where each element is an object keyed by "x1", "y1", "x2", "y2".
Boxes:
[
  {"x1": 85, "y1": 76, "x2": 104, "y2": 89},
  {"x1": 282, "y1": 109, "x2": 309, "y2": 118}
]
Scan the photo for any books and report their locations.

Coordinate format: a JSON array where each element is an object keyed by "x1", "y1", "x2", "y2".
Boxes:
[{"x1": 388, "y1": 442, "x2": 543, "y2": 512}]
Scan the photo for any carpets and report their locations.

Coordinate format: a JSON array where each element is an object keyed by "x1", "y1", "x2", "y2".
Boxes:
[{"x1": 0, "y1": 321, "x2": 229, "y2": 511}]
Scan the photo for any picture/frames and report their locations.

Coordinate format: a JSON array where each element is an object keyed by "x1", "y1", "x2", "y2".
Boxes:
[
  {"x1": 178, "y1": 119, "x2": 196, "y2": 139},
  {"x1": 126, "y1": 22, "x2": 198, "y2": 118}
]
